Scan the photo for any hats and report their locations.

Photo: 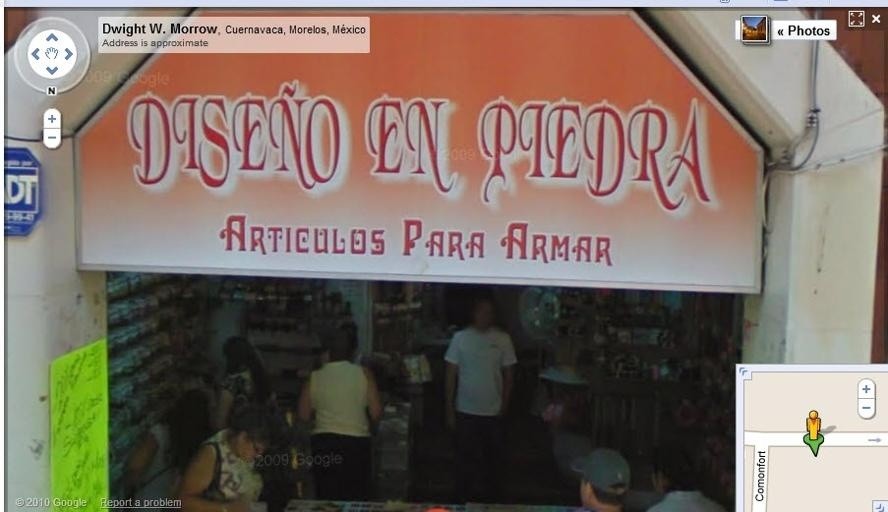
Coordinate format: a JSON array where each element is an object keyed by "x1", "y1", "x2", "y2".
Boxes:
[{"x1": 569, "y1": 448, "x2": 632, "y2": 495}]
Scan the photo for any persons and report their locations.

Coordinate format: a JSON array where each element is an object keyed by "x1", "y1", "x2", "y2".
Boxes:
[
  {"x1": 297, "y1": 338, "x2": 382, "y2": 499},
  {"x1": 128, "y1": 387, "x2": 209, "y2": 472},
  {"x1": 176, "y1": 406, "x2": 276, "y2": 511},
  {"x1": 213, "y1": 337, "x2": 275, "y2": 434},
  {"x1": 571, "y1": 448, "x2": 631, "y2": 511},
  {"x1": 644, "y1": 445, "x2": 726, "y2": 511},
  {"x1": 443, "y1": 297, "x2": 518, "y2": 444}
]
